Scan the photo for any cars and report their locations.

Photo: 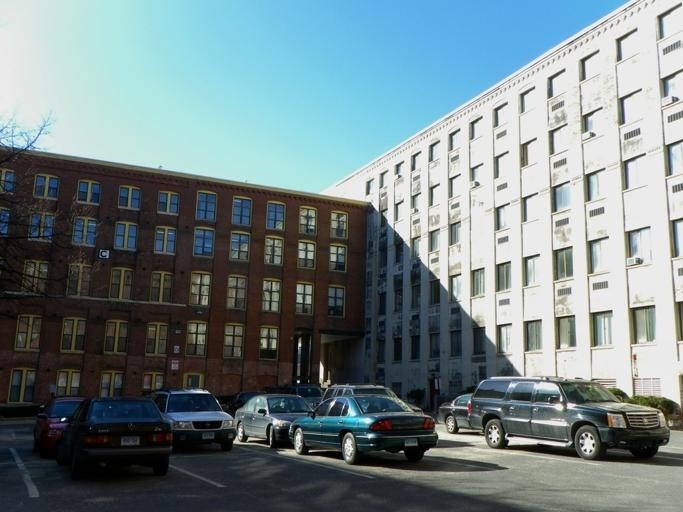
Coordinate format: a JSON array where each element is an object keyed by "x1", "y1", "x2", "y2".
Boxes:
[
  {"x1": 233, "y1": 394, "x2": 313, "y2": 450},
  {"x1": 439, "y1": 393, "x2": 484, "y2": 433},
  {"x1": 228, "y1": 378, "x2": 328, "y2": 411},
  {"x1": 56, "y1": 396, "x2": 169, "y2": 480},
  {"x1": 287, "y1": 393, "x2": 439, "y2": 466},
  {"x1": 32, "y1": 395, "x2": 88, "y2": 459}
]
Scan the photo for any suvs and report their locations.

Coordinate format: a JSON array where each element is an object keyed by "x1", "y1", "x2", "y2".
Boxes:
[
  {"x1": 321, "y1": 384, "x2": 424, "y2": 415},
  {"x1": 467, "y1": 374, "x2": 671, "y2": 460},
  {"x1": 153, "y1": 387, "x2": 236, "y2": 455}
]
